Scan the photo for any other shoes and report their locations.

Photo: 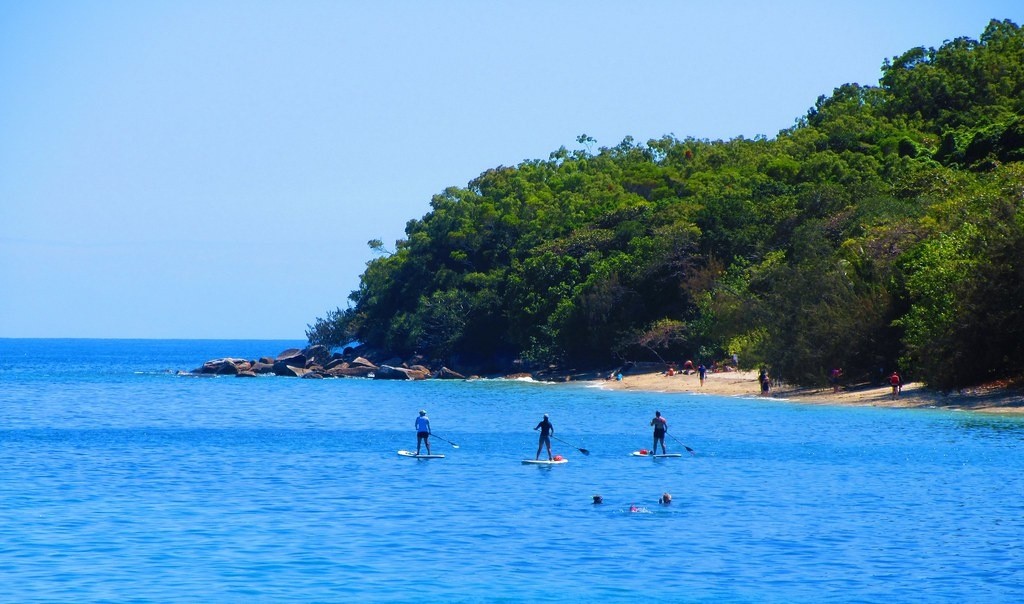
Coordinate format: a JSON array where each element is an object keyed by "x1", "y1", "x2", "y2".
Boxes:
[
  {"x1": 834, "y1": 391, "x2": 839, "y2": 394},
  {"x1": 891, "y1": 397, "x2": 894, "y2": 401},
  {"x1": 896, "y1": 397, "x2": 899, "y2": 400}
]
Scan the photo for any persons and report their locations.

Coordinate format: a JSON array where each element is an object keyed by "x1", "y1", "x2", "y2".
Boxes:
[
  {"x1": 650, "y1": 410, "x2": 668, "y2": 455},
  {"x1": 533, "y1": 413, "x2": 554, "y2": 462},
  {"x1": 658, "y1": 492, "x2": 671, "y2": 504},
  {"x1": 415, "y1": 410, "x2": 431, "y2": 455},
  {"x1": 612, "y1": 351, "x2": 900, "y2": 401}
]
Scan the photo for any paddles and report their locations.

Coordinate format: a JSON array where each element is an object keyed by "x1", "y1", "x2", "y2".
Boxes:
[
  {"x1": 665, "y1": 431, "x2": 696, "y2": 455},
  {"x1": 536, "y1": 429, "x2": 590, "y2": 456},
  {"x1": 429, "y1": 433, "x2": 459, "y2": 449}
]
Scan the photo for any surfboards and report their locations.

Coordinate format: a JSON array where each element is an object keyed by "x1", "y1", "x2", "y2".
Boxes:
[
  {"x1": 397, "y1": 448, "x2": 445, "y2": 458},
  {"x1": 633, "y1": 450, "x2": 682, "y2": 458},
  {"x1": 521, "y1": 458, "x2": 568, "y2": 466}
]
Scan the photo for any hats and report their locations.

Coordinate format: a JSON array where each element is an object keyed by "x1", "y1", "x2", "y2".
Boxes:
[
  {"x1": 420, "y1": 410, "x2": 427, "y2": 416},
  {"x1": 593, "y1": 495, "x2": 602, "y2": 500},
  {"x1": 543, "y1": 414, "x2": 549, "y2": 418},
  {"x1": 630, "y1": 506, "x2": 638, "y2": 512},
  {"x1": 663, "y1": 493, "x2": 671, "y2": 502},
  {"x1": 656, "y1": 411, "x2": 661, "y2": 416}
]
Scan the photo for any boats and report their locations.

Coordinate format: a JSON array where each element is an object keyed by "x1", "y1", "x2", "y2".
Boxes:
[
  {"x1": 633, "y1": 451, "x2": 681, "y2": 457},
  {"x1": 398, "y1": 451, "x2": 444, "y2": 458},
  {"x1": 522, "y1": 457, "x2": 567, "y2": 463}
]
